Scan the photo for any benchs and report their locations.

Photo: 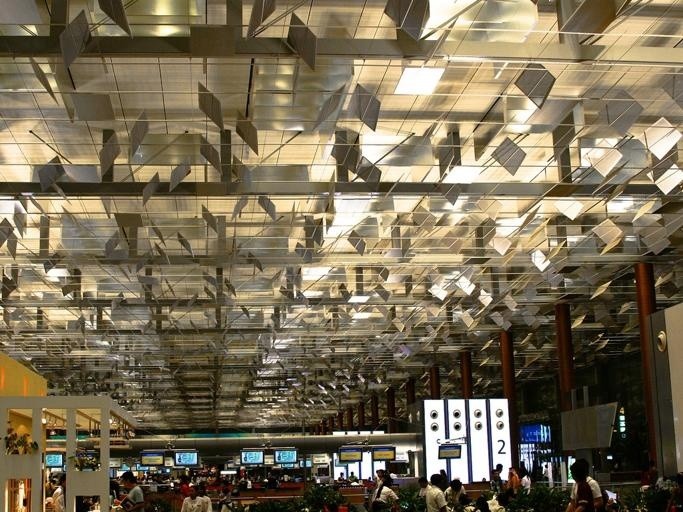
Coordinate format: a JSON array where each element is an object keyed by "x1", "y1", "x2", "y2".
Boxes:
[{"x1": 143, "y1": 483, "x2": 366, "y2": 503}]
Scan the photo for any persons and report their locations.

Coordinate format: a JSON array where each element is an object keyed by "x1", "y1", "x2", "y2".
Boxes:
[{"x1": 51, "y1": 458, "x2": 604, "y2": 512}]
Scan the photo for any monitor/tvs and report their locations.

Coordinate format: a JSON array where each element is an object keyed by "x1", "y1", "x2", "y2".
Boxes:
[
  {"x1": 240, "y1": 449, "x2": 362, "y2": 469},
  {"x1": 438, "y1": 446, "x2": 461, "y2": 459},
  {"x1": 109, "y1": 450, "x2": 198, "y2": 478},
  {"x1": 373, "y1": 447, "x2": 395, "y2": 461},
  {"x1": 46, "y1": 454, "x2": 64, "y2": 467}
]
